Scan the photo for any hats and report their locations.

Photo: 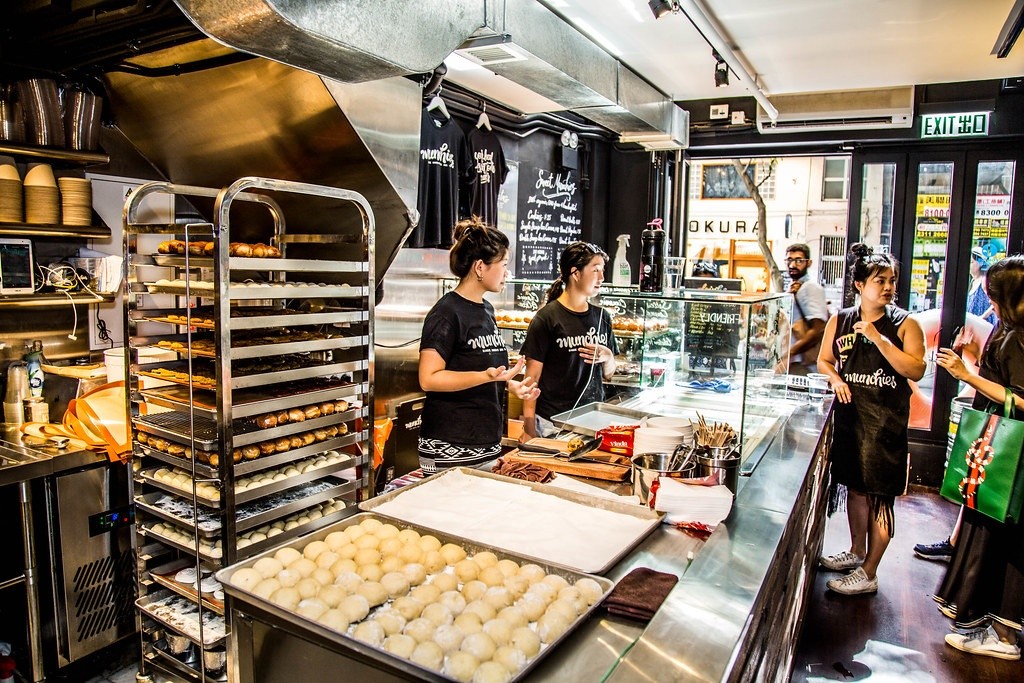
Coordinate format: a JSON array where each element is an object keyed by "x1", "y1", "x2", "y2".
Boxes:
[
  {"x1": 693, "y1": 262, "x2": 719, "y2": 277},
  {"x1": 971, "y1": 247, "x2": 992, "y2": 267}
]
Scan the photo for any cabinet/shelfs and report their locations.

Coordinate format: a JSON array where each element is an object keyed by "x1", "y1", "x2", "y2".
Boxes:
[
  {"x1": 0, "y1": 145, "x2": 115, "y2": 361},
  {"x1": 122, "y1": 176, "x2": 378, "y2": 683},
  {"x1": 438, "y1": 276, "x2": 682, "y2": 391}
]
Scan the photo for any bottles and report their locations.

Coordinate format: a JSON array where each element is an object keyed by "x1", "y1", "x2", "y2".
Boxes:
[
  {"x1": 639, "y1": 218, "x2": 666, "y2": 293},
  {"x1": 26, "y1": 340, "x2": 53, "y2": 398}
]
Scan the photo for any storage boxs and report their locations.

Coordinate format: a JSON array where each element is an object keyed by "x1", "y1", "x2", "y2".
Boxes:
[
  {"x1": 599, "y1": 290, "x2": 794, "y2": 471},
  {"x1": 596, "y1": 424, "x2": 640, "y2": 456}
]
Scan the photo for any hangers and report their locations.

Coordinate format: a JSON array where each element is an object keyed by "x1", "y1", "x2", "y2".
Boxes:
[
  {"x1": 426, "y1": 83, "x2": 451, "y2": 119},
  {"x1": 475, "y1": 101, "x2": 492, "y2": 131}
]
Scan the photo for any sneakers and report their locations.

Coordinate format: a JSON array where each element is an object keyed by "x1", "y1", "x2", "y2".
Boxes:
[
  {"x1": 914, "y1": 536, "x2": 956, "y2": 563},
  {"x1": 944, "y1": 621, "x2": 1023, "y2": 661},
  {"x1": 827, "y1": 565, "x2": 878, "y2": 594},
  {"x1": 821, "y1": 550, "x2": 867, "y2": 571}
]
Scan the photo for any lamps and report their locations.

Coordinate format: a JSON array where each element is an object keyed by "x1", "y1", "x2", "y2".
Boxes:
[
  {"x1": 712, "y1": 48, "x2": 729, "y2": 87},
  {"x1": 648, "y1": 0, "x2": 680, "y2": 20},
  {"x1": 557, "y1": 129, "x2": 579, "y2": 172},
  {"x1": 989, "y1": 0, "x2": 1024, "y2": 59}
]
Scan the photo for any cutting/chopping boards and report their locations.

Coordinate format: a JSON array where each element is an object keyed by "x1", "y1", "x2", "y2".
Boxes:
[{"x1": 504, "y1": 437, "x2": 631, "y2": 481}]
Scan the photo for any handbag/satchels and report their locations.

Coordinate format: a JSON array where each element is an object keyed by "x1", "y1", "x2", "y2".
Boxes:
[
  {"x1": 939, "y1": 385, "x2": 1024, "y2": 526},
  {"x1": 792, "y1": 290, "x2": 832, "y2": 365}
]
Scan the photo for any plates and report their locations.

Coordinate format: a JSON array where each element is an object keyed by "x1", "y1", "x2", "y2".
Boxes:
[
  {"x1": 58, "y1": 177, "x2": 93, "y2": 226},
  {"x1": 630, "y1": 416, "x2": 692, "y2": 483}
]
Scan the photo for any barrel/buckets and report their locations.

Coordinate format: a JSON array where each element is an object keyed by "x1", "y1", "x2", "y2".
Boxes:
[{"x1": 103, "y1": 347, "x2": 177, "y2": 415}]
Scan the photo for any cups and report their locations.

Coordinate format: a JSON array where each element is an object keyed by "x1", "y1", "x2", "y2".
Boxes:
[
  {"x1": 754, "y1": 368, "x2": 776, "y2": 398},
  {"x1": 3, "y1": 366, "x2": 31, "y2": 423},
  {"x1": 663, "y1": 257, "x2": 686, "y2": 294},
  {"x1": 806, "y1": 372, "x2": 830, "y2": 407}
]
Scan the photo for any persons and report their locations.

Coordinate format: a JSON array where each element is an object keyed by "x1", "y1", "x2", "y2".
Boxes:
[
  {"x1": 775, "y1": 244, "x2": 828, "y2": 376},
  {"x1": 518, "y1": 243, "x2": 619, "y2": 449},
  {"x1": 914, "y1": 254, "x2": 1024, "y2": 660},
  {"x1": 418, "y1": 214, "x2": 542, "y2": 478},
  {"x1": 817, "y1": 244, "x2": 927, "y2": 595},
  {"x1": 966, "y1": 246, "x2": 998, "y2": 326},
  {"x1": 686, "y1": 259, "x2": 729, "y2": 368}
]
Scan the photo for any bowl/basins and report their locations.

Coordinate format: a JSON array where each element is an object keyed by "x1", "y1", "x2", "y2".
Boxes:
[
  {"x1": 693, "y1": 450, "x2": 741, "y2": 501},
  {"x1": 631, "y1": 451, "x2": 696, "y2": 507},
  {"x1": 0, "y1": 154, "x2": 60, "y2": 225}
]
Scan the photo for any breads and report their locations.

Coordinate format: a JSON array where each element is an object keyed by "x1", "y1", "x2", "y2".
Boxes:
[
  {"x1": 493, "y1": 309, "x2": 668, "y2": 331},
  {"x1": 229, "y1": 520, "x2": 602, "y2": 683},
  {"x1": 131, "y1": 241, "x2": 350, "y2": 558}
]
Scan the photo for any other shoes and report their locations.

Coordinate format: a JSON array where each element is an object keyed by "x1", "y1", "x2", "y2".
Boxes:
[{"x1": 932, "y1": 592, "x2": 958, "y2": 620}]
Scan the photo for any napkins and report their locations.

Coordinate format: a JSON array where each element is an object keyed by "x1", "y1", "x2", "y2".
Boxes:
[{"x1": 654, "y1": 477, "x2": 734, "y2": 529}]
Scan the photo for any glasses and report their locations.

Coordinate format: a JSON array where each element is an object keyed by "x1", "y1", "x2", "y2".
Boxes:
[
  {"x1": 783, "y1": 259, "x2": 809, "y2": 266},
  {"x1": 693, "y1": 263, "x2": 718, "y2": 273},
  {"x1": 575, "y1": 243, "x2": 604, "y2": 268}
]
